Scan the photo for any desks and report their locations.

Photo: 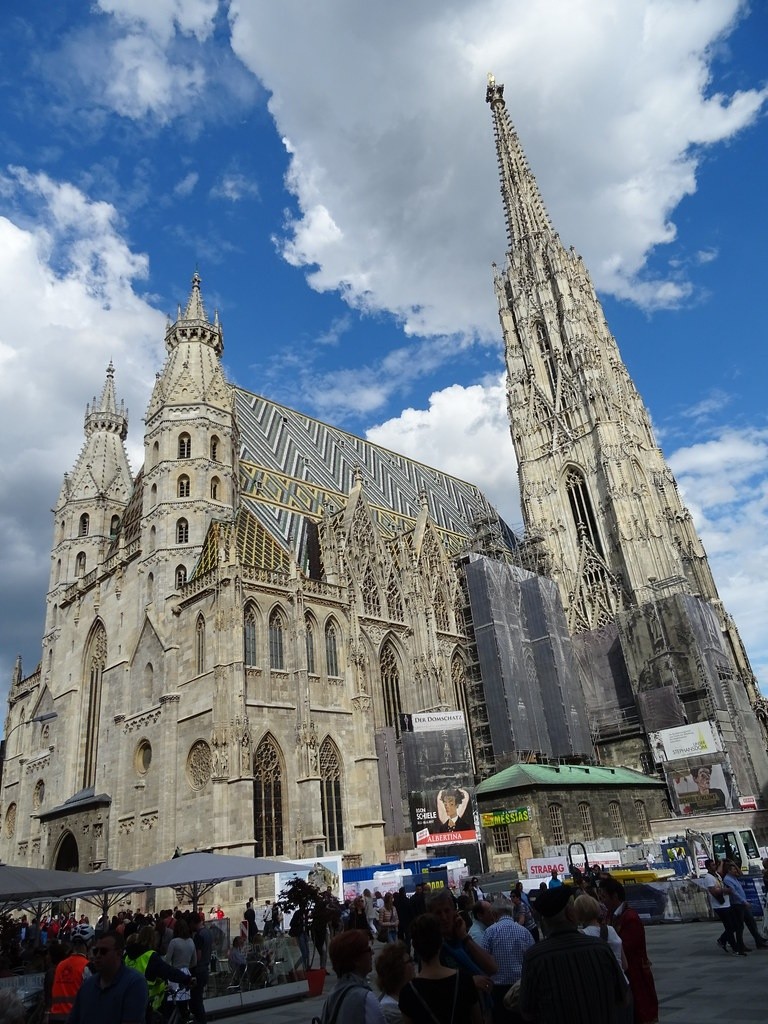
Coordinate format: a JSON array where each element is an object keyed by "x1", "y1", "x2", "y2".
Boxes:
[{"x1": 218, "y1": 958, "x2": 290, "y2": 985}]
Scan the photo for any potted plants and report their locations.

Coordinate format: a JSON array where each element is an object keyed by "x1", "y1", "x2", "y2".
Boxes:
[{"x1": 274, "y1": 876, "x2": 350, "y2": 996}]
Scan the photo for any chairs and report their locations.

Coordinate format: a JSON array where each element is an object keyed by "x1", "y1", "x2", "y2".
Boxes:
[{"x1": 208, "y1": 952, "x2": 274, "y2": 994}]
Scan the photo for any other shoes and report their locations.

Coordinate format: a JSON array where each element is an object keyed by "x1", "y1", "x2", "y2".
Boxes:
[
  {"x1": 740, "y1": 946, "x2": 751, "y2": 952},
  {"x1": 756, "y1": 941, "x2": 768, "y2": 948},
  {"x1": 716, "y1": 939, "x2": 729, "y2": 954},
  {"x1": 732, "y1": 950, "x2": 748, "y2": 957}
]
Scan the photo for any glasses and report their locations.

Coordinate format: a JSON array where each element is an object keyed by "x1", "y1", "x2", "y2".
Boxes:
[
  {"x1": 93, "y1": 946, "x2": 117, "y2": 956},
  {"x1": 510, "y1": 895, "x2": 516, "y2": 898},
  {"x1": 364, "y1": 945, "x2": 372, "y2": 953},
  {"x1": 405, "y1": 956, "x2": 414, "y2": 965},
  {"x1": 360, "y1": 900, "x2": 364, "y2": 902}
]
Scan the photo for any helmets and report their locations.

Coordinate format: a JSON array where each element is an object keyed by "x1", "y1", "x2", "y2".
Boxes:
[{"x1": 70, "y1": 924, "x2": 95, "y2": 945}]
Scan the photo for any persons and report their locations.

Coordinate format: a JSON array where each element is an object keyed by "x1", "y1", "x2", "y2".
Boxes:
[
  {"x1": 0, "y1": 900, "x2": 227, "y2": 1024},
  {"x1": 646, "y1": 850, "x2": 656, "y2": 864},
  {"x1": 373, "y1": 862, "x2": 659, "y2": 1024},
  {"x1": 227, "y1": 880, "x2": 379, "y2": 989},
  {"x1": 703, "y1": 856, "x2": 768, "y2": 957},
  {"x1": 321, "y1": 929, "x2": 388, "y2": 1024}
]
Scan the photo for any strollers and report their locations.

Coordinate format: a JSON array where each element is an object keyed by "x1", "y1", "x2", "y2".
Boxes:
[{"x1": 244, "y1": 952, "x2": 271, "y2": 992}]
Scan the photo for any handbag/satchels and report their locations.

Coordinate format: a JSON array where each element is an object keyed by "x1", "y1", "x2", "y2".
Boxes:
[{"x1": 376, "y1": 928, "x2": 389, "y2": 943}]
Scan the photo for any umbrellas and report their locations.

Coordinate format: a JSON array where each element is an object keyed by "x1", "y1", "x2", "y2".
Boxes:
[
  {"x1": 0, "y1": 865, "x2": 153, "y2": 929},
  {"x1": 59, "y1": 853, "x2": 322, "y2": 911}
]
